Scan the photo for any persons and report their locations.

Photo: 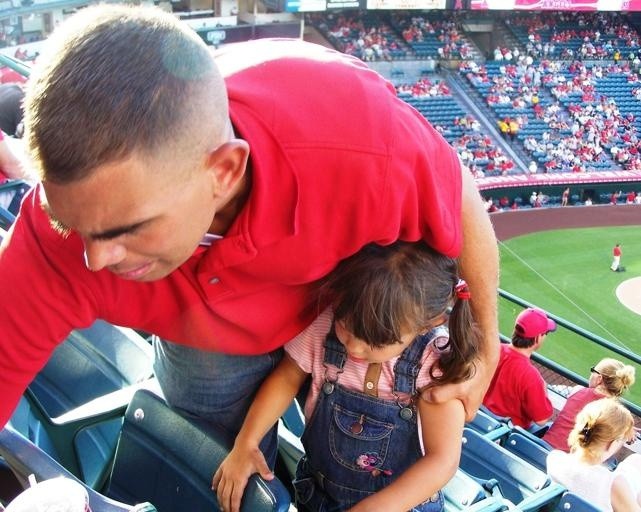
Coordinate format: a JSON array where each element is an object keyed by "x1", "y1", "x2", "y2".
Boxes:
[
  {"x1": 480, "y1": 308, "x2": 641, "y2": 512},
  {"x1": 611, "y1": 245, "x2": 622, "y2": 272},
  {"x1": 305, "y1": 5, "x2": 641, "y2": 212},
  {"x1": 1, "y1": 2, "x2": 502, "y2": 512}
]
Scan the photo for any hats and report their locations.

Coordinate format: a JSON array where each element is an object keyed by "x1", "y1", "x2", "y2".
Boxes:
[{"x1": 515, "y1": 308, "x2": 556, "y2": 338}]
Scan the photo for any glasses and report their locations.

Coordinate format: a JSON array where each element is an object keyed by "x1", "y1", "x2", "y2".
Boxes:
[{"x1": 590, "y1": 367, "x2": 601, "y2": 376}]
